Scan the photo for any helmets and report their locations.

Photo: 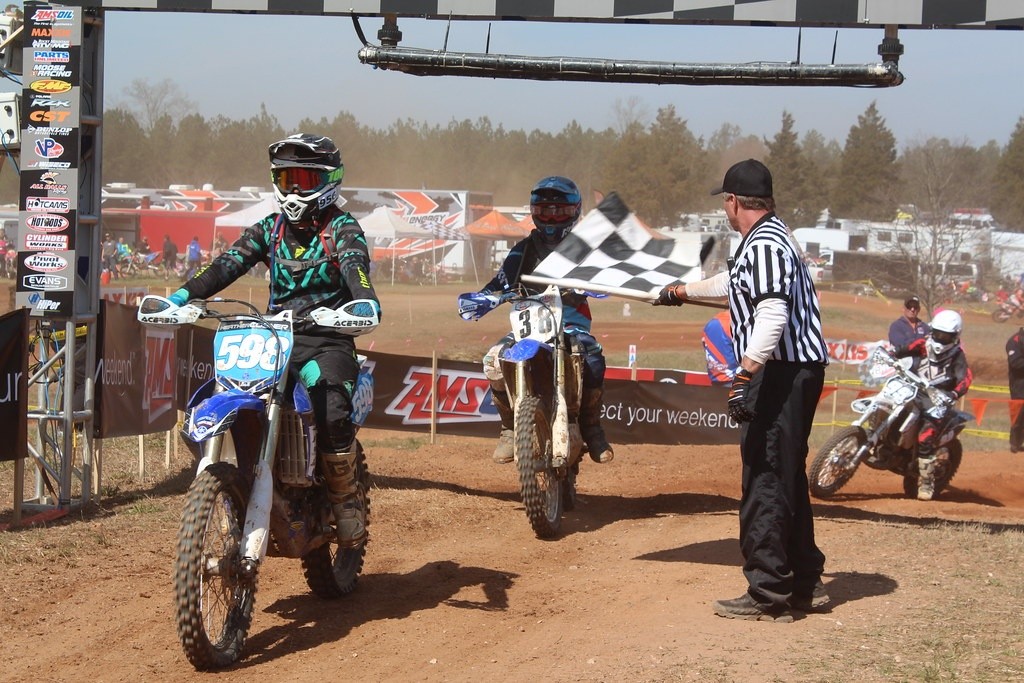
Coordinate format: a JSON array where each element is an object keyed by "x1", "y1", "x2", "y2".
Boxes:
[
  {"x1": 930, "y1": 310, "x2": 961, "y2": 355},
  {"x1": 268, "y1": 133, "x2": 343, "y2": 226},
  {"x1": 529, "y1": 177, "x2": 581, "y2": 243}
]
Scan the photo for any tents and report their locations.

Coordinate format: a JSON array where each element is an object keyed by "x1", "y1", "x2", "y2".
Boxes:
[{"x1": 214, "y1": 197, "x2": 553, "y2": 252}]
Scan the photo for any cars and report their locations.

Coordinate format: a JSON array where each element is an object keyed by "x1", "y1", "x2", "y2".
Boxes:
[{"x1": 801, "y1": 247, "x2": 833, "y2": 284}]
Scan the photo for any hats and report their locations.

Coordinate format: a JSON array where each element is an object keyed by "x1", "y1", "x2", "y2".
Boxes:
[
  {"x1": 711, "y1": 158, "x2": 772, "y2": 198},
  {"x1": 905, "y1": 296, "x2": 920, "y2": 306}
]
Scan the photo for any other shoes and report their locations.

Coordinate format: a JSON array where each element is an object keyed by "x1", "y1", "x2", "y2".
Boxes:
[{"x1": 1011, "y1": 445, "x2": 1024, "y2": 453}]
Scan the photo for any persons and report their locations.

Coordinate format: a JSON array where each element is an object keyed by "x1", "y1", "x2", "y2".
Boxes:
[
  {"x1": 881, "y1": 295, "x2": 973, "y2": 500},
  {"x1": 367, "y1": 255, "x2": 445, "y2": 283},
  {"x1": 653, "y1": 159, "x2": 831, "y2": 622},
  {"x1": 914, "y1": 272, "x2": 1024, "y2": 315},
  {"x1": 159, "y1": 133, "x2": 382, "y2": 547},
  {"x1": 1006, "y1": 326, "x2": 1024, "y2": 453},
  {"x1": 101, "y1": 231, "x2": 229, "y2": 281},
  {"x1": 0, "y1": 228, "x2": 19, "y2": 280},
  {"x1": 468, "y1": 177, "x2": 615, "y2": 464}
]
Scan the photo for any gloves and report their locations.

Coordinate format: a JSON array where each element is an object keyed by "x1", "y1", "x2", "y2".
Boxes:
[
  {"x1": 652, "y1": 284, "x2": 684, "y2": 307},
  {"x1": 909, "y1": 337, "x2": 926, "y2": 351},
  {"x1": 354, "y1": 299, "x2": 382, "y2": 322},
  {"x1": 920, "y1": 343, "x2": 928, "y2": 358},
  {"x1": 727, "y1": 366, "x2": 755, "y2": 425},
  {"x1": 158, "y1": 288, "x2": 189, "y2": 310}
]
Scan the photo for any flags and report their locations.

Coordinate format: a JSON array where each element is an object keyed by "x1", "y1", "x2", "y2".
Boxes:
[{"x1": 521, "y1": 189, "x2": 718, "y2": 305}]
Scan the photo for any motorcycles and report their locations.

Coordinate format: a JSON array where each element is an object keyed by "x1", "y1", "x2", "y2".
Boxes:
[
  {"x1": 993, "y1": 300, "x2": 1023, "y2": 322},
  {"x1": 809, "y1": 345, "x2": 976, "y2": 498},
  {"x1": 133, "y1": 298, "x2": 371, "y2": 671},
  {"x1": 457, "y1": 282, "x2": 596, "y2": 538},
  {"x1": 120, "y1": 241, "x2": 185, "y2": 281}
]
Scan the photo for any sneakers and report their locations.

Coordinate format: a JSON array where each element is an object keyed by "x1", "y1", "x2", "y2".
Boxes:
[
  {"x1": 714, "y1": 592, "x2": 807, "y2": 622},
  {"x1": 793, "y1": 572, "x2": 831, "y2": 611}
]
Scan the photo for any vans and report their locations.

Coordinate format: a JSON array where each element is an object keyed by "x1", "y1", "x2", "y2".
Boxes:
[
  {"x1": 921, "y1": 260, "x2": 984, "y2": 289},
  {"x1": 672, "y1": 221, "x2": 735, "y2": 232}
]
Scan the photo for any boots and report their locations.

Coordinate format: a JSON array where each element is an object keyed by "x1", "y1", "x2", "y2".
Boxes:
[
  {"x1": 917, "y1": 455, "x2": 937, "y2": 500},
  {"x1": 316, "y1": 430, "x2": 366, "y2": 548},
  {"x1": 489, "y1": 382, "x2": 515, "y2": 464},
  {"x1": 221, "y1": 514, "x2": 229, "y2": 535},
  {"x1": 579, "y1": 385, "x2": 615, "y2": 463}
]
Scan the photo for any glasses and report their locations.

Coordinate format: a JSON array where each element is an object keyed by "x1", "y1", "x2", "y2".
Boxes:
[
  {"x1": 932, "y1": 329, "x2": 957, "y2": 346},
  {"x1": 534, "y1": 202, "x2": 577, "y2": 224},
  {"x1": 905, "y1": 304, "x2": 920, "y2": 310},
  {"x1": 270, "y1": 162, "x2": 329, "y2": 197}
]
